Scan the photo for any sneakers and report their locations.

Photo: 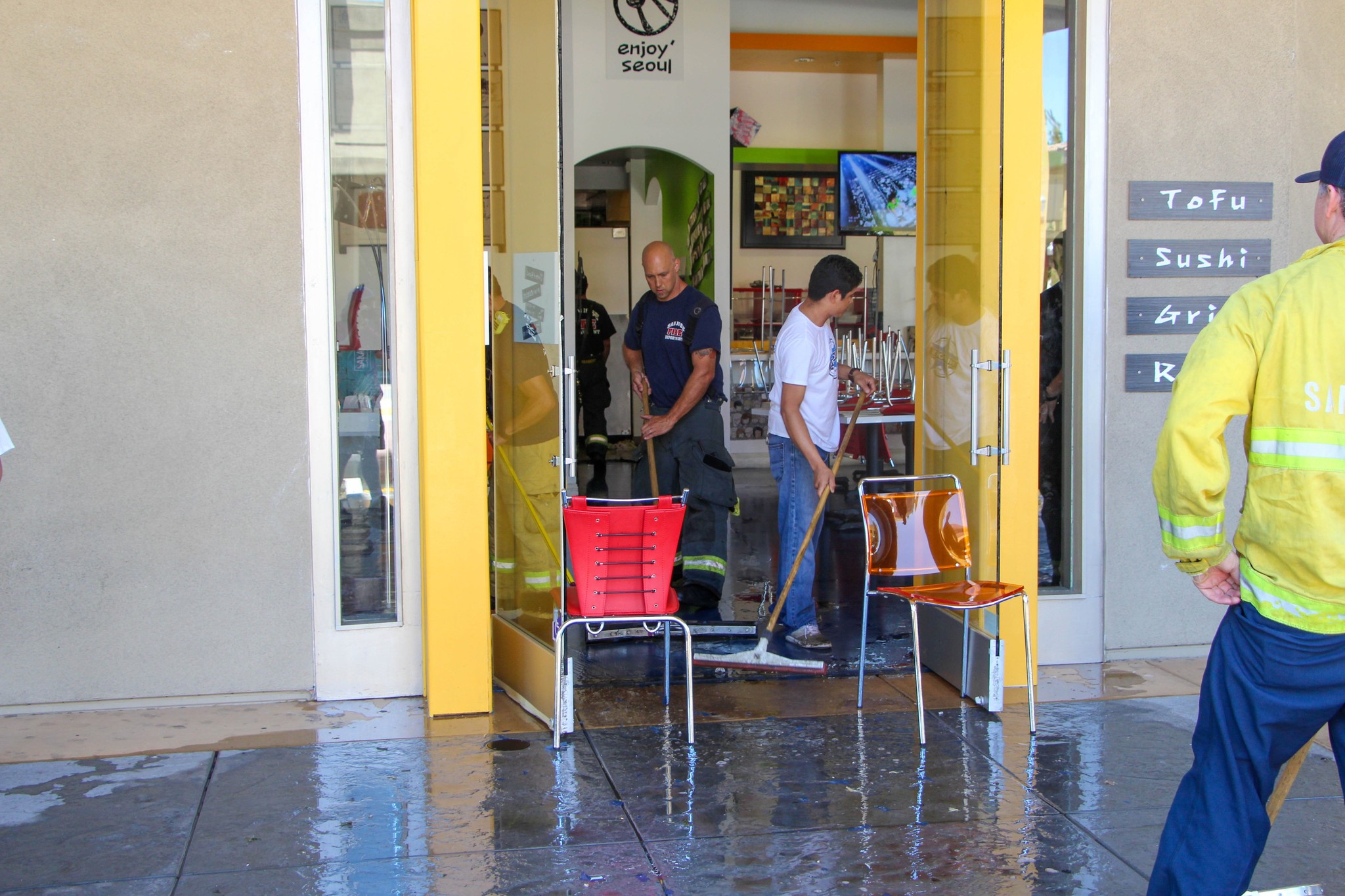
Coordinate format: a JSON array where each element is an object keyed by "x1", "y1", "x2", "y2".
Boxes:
[{"x1": 785, "y1": 625, "x2": 833, "y2": 648}]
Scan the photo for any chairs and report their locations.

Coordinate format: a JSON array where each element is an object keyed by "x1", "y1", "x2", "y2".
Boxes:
[
  {"x1": 858, "y1": 472, "x2": 1038, "y2": 747},
  {"x1": 550, "y1": 488, "x2": 694, "y2": 749},
  {"x1": 750, "y1": 326, "x2": 916, "y2": 468}
]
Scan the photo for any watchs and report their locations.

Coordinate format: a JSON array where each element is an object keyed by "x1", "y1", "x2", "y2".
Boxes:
[
  {"x1": 1043, "y1": 388, "x2": 1060, "y2": 402},
  {"x1": 848, "y1": 367, "x2": 863, "y2": 383}
]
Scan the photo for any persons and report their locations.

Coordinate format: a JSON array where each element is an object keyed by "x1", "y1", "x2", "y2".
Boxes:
[
  {"x1": 486, "y1": 268, "x2": 561, "y2": 620},
  {"x1": 1144, "y1": 132, "x2": 1345, "y2": 896},
  {"x1": 767, "y1": 254, "x2": 878, "y2": 648},
  {"x1": 920, "y1": 252, "x2": 1006, "y2": 589},
  {"x1": 1041, "y1": 232, "x2": 1067, "y2": 588},
  {"x1": 575, "y1": 271, "x2": 616, "y2": 492},
  {"x1": 622, "y1": 241, "x2": 738, "y2": 620}
]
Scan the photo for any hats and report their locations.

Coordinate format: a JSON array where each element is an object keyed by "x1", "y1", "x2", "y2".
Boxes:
[{"x1": 1294, "y1": 129, "x2": 1345, "y2": 188}]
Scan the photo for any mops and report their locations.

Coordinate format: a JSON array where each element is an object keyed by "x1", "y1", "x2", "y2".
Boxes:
[
  {"x1": 693, "y1": 389, "x2": 865, "y2": 674},
  {"x1": 584, "y1": 378, "x2": 759, "y2": 643}
]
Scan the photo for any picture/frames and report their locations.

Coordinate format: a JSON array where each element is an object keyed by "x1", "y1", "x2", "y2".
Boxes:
[{"x1": 742, "y1": 162, "x2": 845, "y2": 249}]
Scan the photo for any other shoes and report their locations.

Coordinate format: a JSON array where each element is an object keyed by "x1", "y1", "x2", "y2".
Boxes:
[{"x1": 585, "y1": 479, "x2": 609, "y2": 493}]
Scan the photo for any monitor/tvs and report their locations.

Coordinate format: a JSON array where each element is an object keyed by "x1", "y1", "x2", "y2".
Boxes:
[{"x1": 838, "y1": 150, "x2": 916, "y2": 236}]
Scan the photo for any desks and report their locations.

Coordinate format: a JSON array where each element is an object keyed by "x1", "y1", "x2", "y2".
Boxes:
[{"x1": 752, "y1": 408, "x2": 914, "y2": 554}]
